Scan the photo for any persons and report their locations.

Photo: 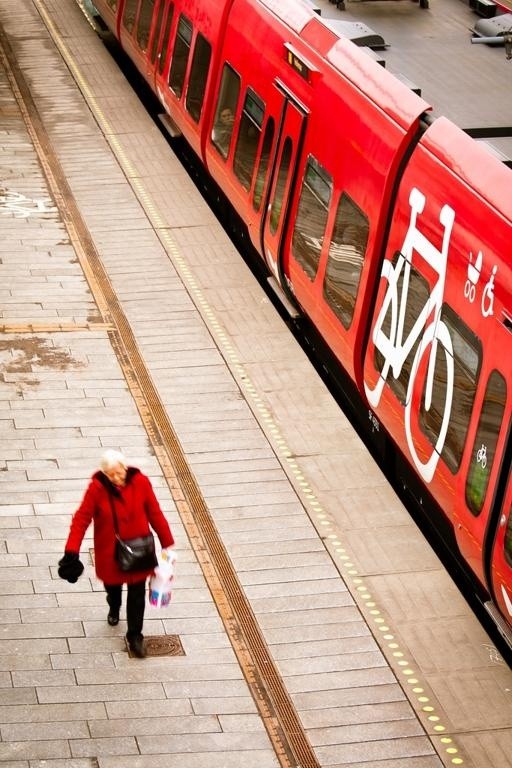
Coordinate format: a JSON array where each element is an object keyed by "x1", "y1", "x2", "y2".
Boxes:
[
  {"x1": 56, "y1": 449, "x2": 177, "y2": 659},
  {"x1": 213, "y1": 107, "x2": 259, "y2": 156}
]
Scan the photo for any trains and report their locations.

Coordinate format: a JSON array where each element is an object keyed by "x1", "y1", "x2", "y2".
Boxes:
[{"x1": 90, "y1": 1, "x2": 512, "y2": 649}]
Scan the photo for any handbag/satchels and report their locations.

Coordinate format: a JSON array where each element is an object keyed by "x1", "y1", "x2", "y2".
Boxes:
[{"x1": 113, "y1": 535, "x2": 159, "y2": 572}]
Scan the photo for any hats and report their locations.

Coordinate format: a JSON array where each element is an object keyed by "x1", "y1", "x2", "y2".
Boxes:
[{"x1": 58, "y1": 552, "x2": 84, "y2": 583}]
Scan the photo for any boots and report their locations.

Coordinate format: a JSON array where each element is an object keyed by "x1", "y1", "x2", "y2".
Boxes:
[
  {"x1": 107, "y1": 604, "x2": 120, "y2": 625},
  {"x1": 126, "y1": 631, "x2": 147, "y2": 658}
]
